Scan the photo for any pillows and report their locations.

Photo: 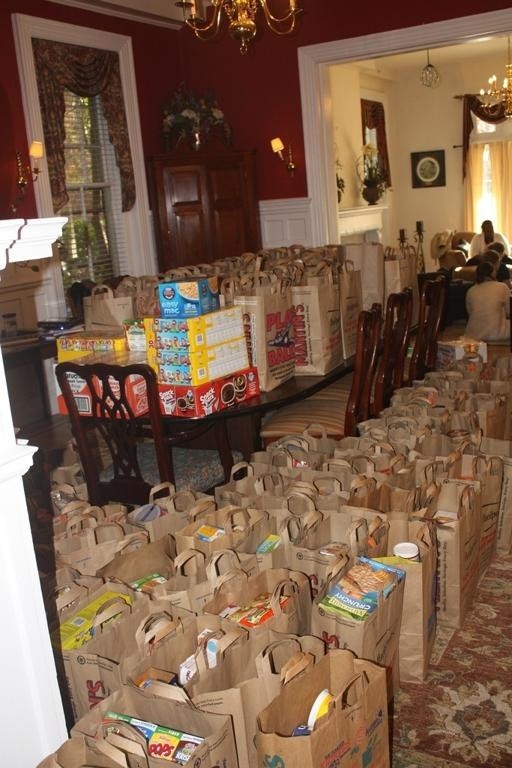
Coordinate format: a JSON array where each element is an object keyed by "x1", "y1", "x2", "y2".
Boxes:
[{"x1": 456, "y1": 237, "x2": 470, "y2": 255}]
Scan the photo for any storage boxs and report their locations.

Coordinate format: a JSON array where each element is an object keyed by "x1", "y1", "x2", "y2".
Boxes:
[
  {"x1": 437, "y1": 341, "x2": 489, "y2": 366},
  {"x1": 55, "y1": 277, "x2": 260, "y2": 420}
]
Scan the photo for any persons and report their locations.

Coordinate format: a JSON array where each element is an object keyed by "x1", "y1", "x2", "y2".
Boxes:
[
  {"x1": 487, "y1": 241, "x2": 512, "y2": 289},
  {"x1": 468, "y1": 220, "x2": 510, "y2": 261},
  {"x1": 482, "y1": 249, "x2": 500, "y2": 271},
  {"x1": 464, "y1": 261, "x2": 512, "y2": 342}
]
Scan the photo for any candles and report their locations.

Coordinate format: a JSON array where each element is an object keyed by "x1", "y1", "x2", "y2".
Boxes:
[
  {"x1": 400, "y1": 229, "x2": 404, "y2": 239},
  {"x1": 416, "y1": 219, "x2": 423, "y2": 231}
]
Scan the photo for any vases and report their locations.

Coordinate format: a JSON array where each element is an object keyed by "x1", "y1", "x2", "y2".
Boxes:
[
  {"x1": 336, "y1": 190, "x2": 341, "y2": 203},
  {"x1": 362, "y1": 186, "x2": 382, "y2": 205},
  {"x1": 187, "y1": 128, "x2": 206, "y2": 152}
]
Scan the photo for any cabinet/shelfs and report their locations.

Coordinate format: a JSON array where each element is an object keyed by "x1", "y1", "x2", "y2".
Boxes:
[
  {"x1": 260, "y1": 198, "x2": 312, "y2": 252},
  {"x1": 154, "y1": 146, "x2": 256, "y2": 266}
]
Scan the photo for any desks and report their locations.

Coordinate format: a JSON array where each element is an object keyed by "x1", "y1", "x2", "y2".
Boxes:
[{"x1": 80, "y1": 317, "x2": 432, "y2": 498}]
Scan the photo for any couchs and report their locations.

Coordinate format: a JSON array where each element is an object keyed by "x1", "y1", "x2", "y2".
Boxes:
[
  {"x1": 452, "y1": 265, "x2": 512, "y2": 281},
  {"x1": 439, "y1": 233, "x2": 477, "y2": 271}
]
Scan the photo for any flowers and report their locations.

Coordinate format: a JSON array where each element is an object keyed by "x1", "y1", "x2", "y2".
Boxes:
[
  {"x1": 332, "y1": 157, "x2": 346, "y2": 193},
  {"x1": 162, "y1": 93, "x2": 229, "y2": 133},
  {"x1": 354, "y1": 144, "x2": 390, "y2": 190}
]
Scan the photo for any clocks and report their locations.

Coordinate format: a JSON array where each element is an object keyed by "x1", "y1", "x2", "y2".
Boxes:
[{"x1": 410, "y1": 150, "x2": 446, "y2": 188}]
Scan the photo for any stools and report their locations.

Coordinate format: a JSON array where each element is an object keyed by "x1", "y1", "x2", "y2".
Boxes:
[{"x1": 465, "y1": 334, "x2": 512, "y2": 364}]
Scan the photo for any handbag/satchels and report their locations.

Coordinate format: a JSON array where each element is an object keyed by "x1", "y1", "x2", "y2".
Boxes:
[
  {"x1": 81, "y1": 290, "x2": 139, "y2": 332},
  {"x1": 115, "y1": 244, "x2": 351, "y2": 316},
  {"x1": 70, "y1": 684, "x2": 241, "y2": 767},
  {"x1": 255, "y1": 647, "x2": 394, "y2": 767},
  {"x1": 27, "y1": 494, "x2": 127, "y2": 550},
  {"x1": 447, "y1": 457, "x2": 504, "y2": 582},
  {"x1": 310, "y1": 555, "x2": 408, "y2": 706},
  {"x1": 362, "y1": 516, "x2": 441, "y2": 686},
  {"x1": 337, "y1": 271, "x2": 367, "y2": 361},
  {"x1": 232, "y1": 273, "x2": 297, "y2": 393},
  {"x1": 290, "y1": 511, "x2": 373, "y2": 601},
  {"x1": 255, "y1": 477, "x2": 315, "y2": 534},
  {"x1": 95, "y1": 532, "x2": 213, "y2": 600},
  {"x1": 288, "y1": 284, "x2": 345, "y2": 378},
  {"x1": 420, "y1": 478, "x2": 484, "y2": 630},
  {"x1": 355, "y1": 357, "x2": 510, "y2": 473},
  {"x1": 33, "y1": 733, "x2": 128, "y2": 768},
  {"x1": 48, "y1": 579, "x2": 154, "y2": 722},
  {"x1": 48, "y1": 521, "x2": 150, "y2": 574},
  {"x1": 183, "y1": 626, "x2": 329, "y2": 767},
  {"x1": 40, "y1": 565, "x2": 105, "y2": 621},
  {"x1": 173, "y1": 502, "x2": 269, "y2": 552},
  {"x1": 152, "y1": 549, "x2": 260, "y2": 611},
  {"x1": 382, "y1": 254, "x2": 421, "y2": 331},
  {"x1": 214, "y1": 470, "x2": 286, "y2": 510},
  {"x1": 125, "y1": 489, "x2": 217, "y2": 543},
  {"x1": 69, "y1": 596, "x2": 201, "y2": 720},
  {"x1": 201, "y1": 567, "x2": 314, "y2": 636},
  {"x1": 245, "y1": 428, "x2": 455, "y2": 523},
  {"x1": 123, "y1": 614, "x2": 250, "y2": 704},
  {"x1": 233, "y1": 514, "x2": 296, "y2": 569}
]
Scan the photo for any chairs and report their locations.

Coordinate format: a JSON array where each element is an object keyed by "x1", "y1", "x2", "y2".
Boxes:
[
  {"x1": 54, "y1": 364, "x2": 177, "y2": 510},
  {"x1": 260, "y1": 268, "x2": 452, "y2": 452}
]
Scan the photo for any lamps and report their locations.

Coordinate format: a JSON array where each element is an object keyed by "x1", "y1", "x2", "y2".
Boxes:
[
  {"x1": 270, "y1": 137, "x2": 298, "y2": 177},
  {"x1": 480, "y1": 35, "x2": 512, "y2": 117},
  {"x1": 173, "y1": 0, "x2": 303, "y2": 55},
  {"x1": 420, "y1": 49, "x2": 439, "y2": 88},
  {"x1": 7, "y1": 141, "x2": 44, "y2": 216}
]
